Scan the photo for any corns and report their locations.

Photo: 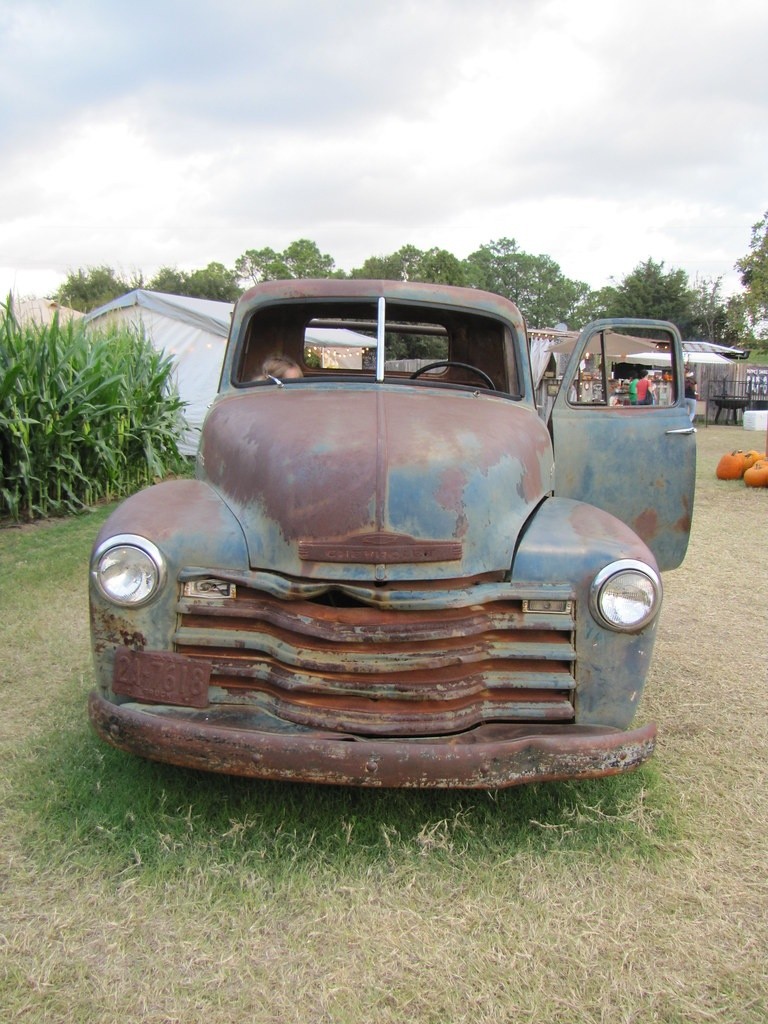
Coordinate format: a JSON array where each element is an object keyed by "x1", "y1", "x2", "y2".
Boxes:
[{"x1": 9, "y1": 415, "x2": 125, "y2": 443}]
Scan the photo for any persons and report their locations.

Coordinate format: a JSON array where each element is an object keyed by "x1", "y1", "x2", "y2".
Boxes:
[
  {"x1": 261, "y1": 351, "x2": 304, "y2": 379},
  {"x1": 635, "y1": 371, "x2": 654, "y2": 406},
  {"x1": 628, "y1": 372, "x2": 639, "y2": 405},
  {"x1": 685, "y1": 368, "x2": 698, "y2": 423}
]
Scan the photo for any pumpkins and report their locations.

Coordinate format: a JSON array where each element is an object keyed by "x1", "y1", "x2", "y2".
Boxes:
[{"x1": 716, "y1": 450, "x2": 768, "y2": 488}]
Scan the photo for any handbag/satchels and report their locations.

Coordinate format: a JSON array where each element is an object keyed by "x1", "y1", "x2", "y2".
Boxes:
[{"x1": 645, "y1": 389, "x2": 653, "y2": 405}]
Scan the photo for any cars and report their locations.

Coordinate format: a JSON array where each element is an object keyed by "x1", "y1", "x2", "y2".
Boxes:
[{"x1": 78, "y1": 278, "x2": 704, "y2": 791}]
